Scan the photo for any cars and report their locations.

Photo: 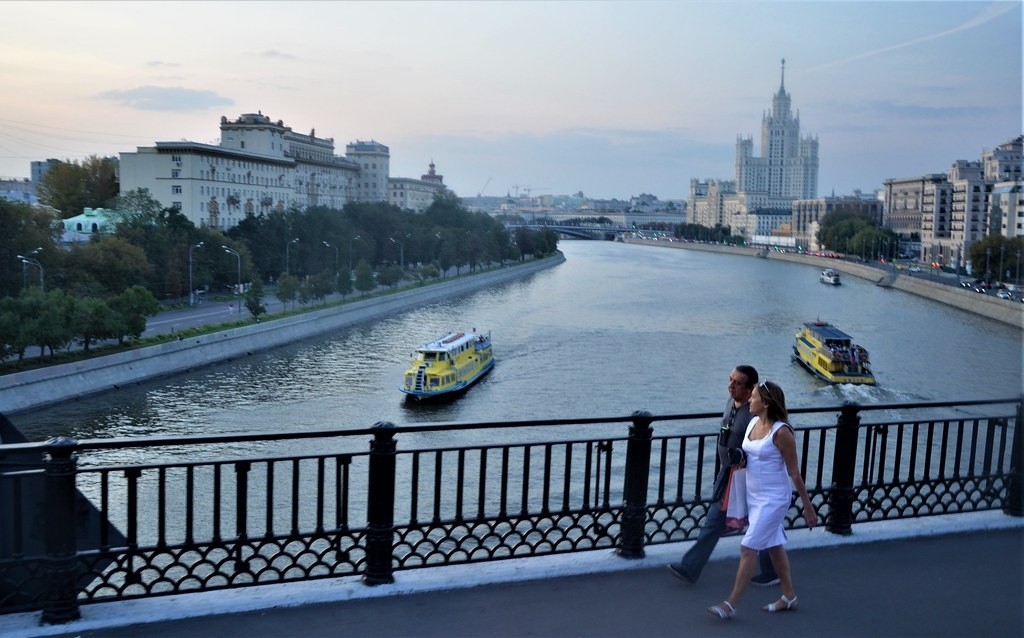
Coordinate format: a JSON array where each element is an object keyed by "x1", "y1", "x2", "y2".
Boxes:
[{"x1": 959, "y1": 278, "x2": 1024, "y2": 304}]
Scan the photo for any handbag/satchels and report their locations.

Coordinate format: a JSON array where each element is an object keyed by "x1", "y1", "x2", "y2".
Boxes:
[{"x1": 720, "y1": 466, "x2": 749, "y2": 529}]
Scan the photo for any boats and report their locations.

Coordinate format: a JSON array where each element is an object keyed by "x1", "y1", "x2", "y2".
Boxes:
[
  {"x1": 398, "y1": 326, "x2": 496, "y2": 402},
  {"x1": 819, "y1": 268, "x2": 843, "y2": 286},
  {"x1": 791, "y1": 314, "x2": 876, "y2": 387}
]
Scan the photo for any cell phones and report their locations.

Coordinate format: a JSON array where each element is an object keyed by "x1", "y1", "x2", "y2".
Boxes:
[{"x1": 719, "y1": 426, "x2": 729, "y2": 447}]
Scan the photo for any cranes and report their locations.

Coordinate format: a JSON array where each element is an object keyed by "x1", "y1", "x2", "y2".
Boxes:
[
  {"x1": 512, "y1": 183, "x2": 552, "y2": 199},
  {"x1": 476, "y1": 176, "x2": 492, "y2": 198}
]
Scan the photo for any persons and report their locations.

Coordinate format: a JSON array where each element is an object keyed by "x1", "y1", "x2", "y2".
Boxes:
[
  {"x1": 706, "y1": 376, "x2": 818, "y2": 622},
  {"x1": 229, "y1": 301, "x2": 234, "y2": 315},
  {"x1": 828, "y1": 342, "x2": 869, "y2": 374},
  {"x1": 668, "y1": 365, "x2": 781, "y2": 586}
]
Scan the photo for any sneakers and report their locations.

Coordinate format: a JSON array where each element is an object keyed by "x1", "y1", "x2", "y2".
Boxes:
[
  {"x1": 750, "y1": 572, "x2": 780, "y2": 585},
  {"x1": 667, "y1": 563, "x2": 697, "y2": 585}
]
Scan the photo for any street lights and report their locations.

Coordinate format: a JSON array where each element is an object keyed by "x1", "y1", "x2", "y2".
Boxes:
[
  {"x1": 322, "y1": 240, "x2": 338, "y2": 277},
  {"x1": 189, "y1": 242, "x2": 205, "y2": 307},
  {"x1": 984, "y1": 247, "x2": 991, "y2": 295},
  {"x1": 22, "y1": 247, "x2": 43, "y2": 290},
  {"x1": 1015, "y1": 249, "x2": 1021, "y2": 284},
  {"x1": 286, "y1": 237, "x2": 300, "y2": 274},
  {"x1": 15, "y1": 254, "x2": 45, "y2": 292},
  {"x1": 390, "y1": 237, "x2": 403, "y2": 271},
  {"x1": 221, "y1": 245, "x2": 242, "y2": 321},
  {"x1": 999, "y1": 244, "x2": 1005, "y2": 284},
  {"x1": 349, "y1": 235, "x2": 360, "y2": 273}
]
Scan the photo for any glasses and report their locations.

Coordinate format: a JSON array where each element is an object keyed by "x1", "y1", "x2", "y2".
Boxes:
[{"x1": 759, "y1": 377, "x2": 770, "y2": 392}]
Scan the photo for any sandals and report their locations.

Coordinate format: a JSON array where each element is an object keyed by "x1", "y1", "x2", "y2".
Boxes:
[
  {"x1": 706, "y1": 600, "x2": 737, "y2": 619},
  {"x1": 763, "y1": 594, "x2": 800, "y2": 612}
]
